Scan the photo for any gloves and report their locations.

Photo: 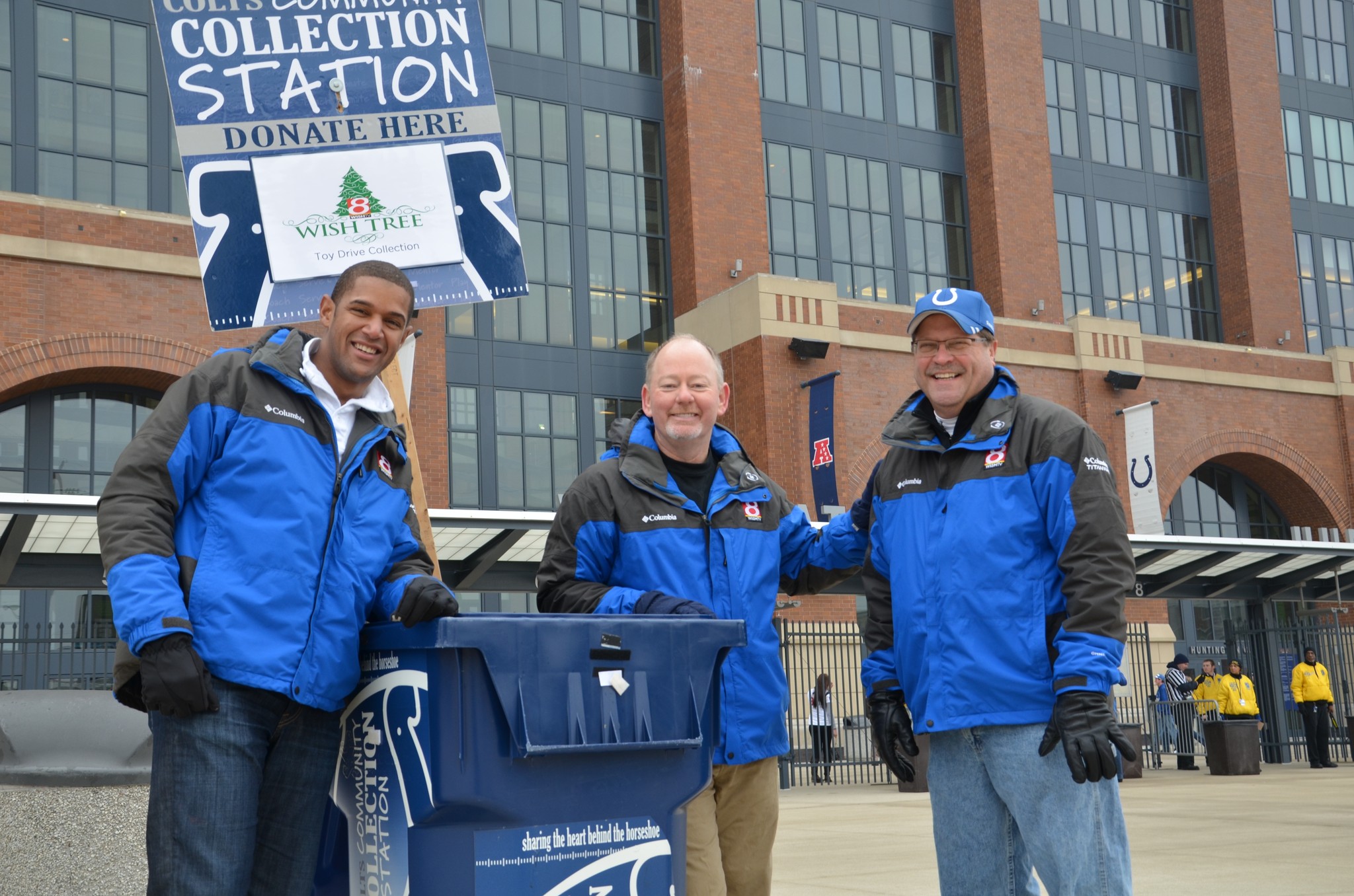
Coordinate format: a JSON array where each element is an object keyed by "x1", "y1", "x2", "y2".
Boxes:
[
  {"x1": 1196, "y1": 674, "x2": 1205, "y2": 685},
  {"x1": 1298, "y1": 707, "x2": 1308, "y2": 716},
  {"x1": 393, "y1": 581, "x2": 459, "y2": 629},
  {"x1": 139, "y1": 631, "x2": 219, "y2": 717},
  {"x1": 852, "y1": 458, "x2": 882, "y2": 530},
  {"x1": 867, "y1": 692, "x2": 920, "y2": 784},
  {"x1": 1037, "y1": 689, "x2": 1138, "y2": 784},
  {"x1": 633, "y1": 592, "x2": 716, "y2": 619}
]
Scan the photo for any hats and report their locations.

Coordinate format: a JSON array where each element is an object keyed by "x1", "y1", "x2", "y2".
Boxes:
[
  {"x1": 907, "y1": 288, "x2": 993, "y2": 335},
  {"x1": 1154, "y1": 674, "x2": 1165, "y2": 681},
  {"x1": 1304, "y1": 646, "x2": 1317, "y2": 656},
  {"x1": 1229, "y1": 659, "x2": 1242, "y2": 669},
  {"x1": 1174, "y1": 653, "x2": 1189, "y2": 665}
]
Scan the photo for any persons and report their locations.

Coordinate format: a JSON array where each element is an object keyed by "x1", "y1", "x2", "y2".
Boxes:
[
  {"x1": 860, "y1": 287, "x2": 1137, "y2": 896},
  {"x1": 1150, "y1": 653, "x2": 1261, "y2": 772},
  {"x1": 97, "y1": 259, "x2": 457, "y2": 896},
  {"x1": 537, "y1": 332, "x2": 885, "y2": 896},
  {"x1": 807, "y1": 674, "x2": 837, "y2": 783},
  {"x1": 1290, "y1": 646, "x2": 1338, "y2": 768}
]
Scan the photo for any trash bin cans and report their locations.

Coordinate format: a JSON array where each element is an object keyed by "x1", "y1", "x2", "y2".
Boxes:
[
  {"x1": 329, "y1": 615, "x2": 746, "y2": 896},
  {"x1": 1345, "y1": 715, "x2": 1354, "y2": 763},
  {"x1": 1203, "y1": 719, "x2": 1261, "y2": 776},
  {"x1": 1118, "y1": 723, "x2": 1144, "y2": 778},
  {"x1": 843, "y1": 715, "x2": 871, "y2": 760}
]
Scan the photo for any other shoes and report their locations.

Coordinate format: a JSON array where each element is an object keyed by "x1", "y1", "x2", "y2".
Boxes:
[
  {"x1": 812, "y1": 774, "x2": 825, "y2": 783},
  {"x1": 825, "y1": 775, "x2": 832, "y2": 783},
  {"x1": 1310, "y1": 761, "x2": 1324, "y2": 769},
  {"x1": 1178, "y1": 764, "x2": 1200, "y2": 771},
  {"x1": 1322, "y1": 761, "x2": 1338, "y2": 768}
]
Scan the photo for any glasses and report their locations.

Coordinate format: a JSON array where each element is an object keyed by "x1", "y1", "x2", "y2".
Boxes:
[{"x1": 910, "y1": 338, "x2": 988, "y2": 359}]
